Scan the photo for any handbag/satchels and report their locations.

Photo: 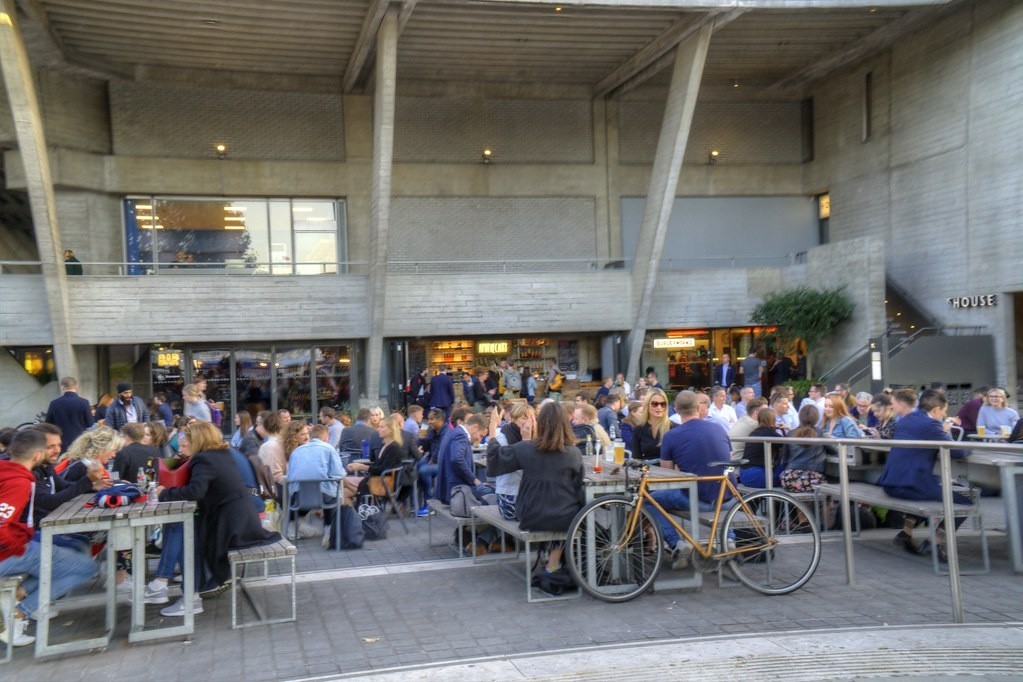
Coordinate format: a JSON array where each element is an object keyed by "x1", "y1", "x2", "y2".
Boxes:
[
  {"x1": 448, "y1": 481, "x2": 495, "y2": 517},
  {"x1": 467, "y1": 542, "x2": 488, "y2": 556},
  {"x1": 155, "y1": 452, "x2": 189, "y2": 489},
  {"x1": 571, "y1": 424, "x2": 594, "y2": 455},
  {"x1": 208, "y1": 399, "x2": 222, "y2": 426},
  {"x1": 835, "y1": 505, "x2": 876, "y2": 529}
]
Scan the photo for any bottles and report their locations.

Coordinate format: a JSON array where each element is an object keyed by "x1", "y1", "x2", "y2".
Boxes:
[
  {"x1": 145, "y1": 461, "x2": 156, "y2": 484},
  {"x1": 615, "y1": 436, "x2": 623, "y2": 442},
  {"x1": 362, "y1": 440, "x2": 369, "y2": 459},
  {"x1": 610, "y1": 425, "x2": 616, "y2": 441},
  {"x1": 137, "y1": 467, "x2": 146, "y2": 489},
  {"x1": 593, "y1": 439, "x2": 603, "y2": 473},
  {"x1": 146, "y1": 482, "x2": 158, "y2": 505},
  {"x1": 585, "y1": 434, "x2": 593, "y2": 457}
]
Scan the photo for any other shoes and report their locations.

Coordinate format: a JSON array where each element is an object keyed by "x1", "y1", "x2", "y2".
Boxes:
[
  {"x1": 918, "y1": 540, "x2": 947, "y2": 562},
  {"x1": 200, "y1": 576, "x2": 240, "y2": 597},
  {"x1": 418, "y1": 505, "x2": 436, "y2": 517},
  {"x1": 489, "y1": 544, "x2": 515, "y2": 552},
  {"x1": 124, "y1": 540, "x2": 161, "y2": 559},
  {"x1": 671, "y1": 539, "x2": 693, "y2": 570},
  {"x1": 16, "y1": 596, "x2": 58, "y2": 619},
  {"x1": 895, "y1": 529, "x2": 919, "y2": 554},
  {"x1": 105, "y1": 571, "x2": 147, "y2": 592},
  {"x1": 0, "y1": 592, "x2": 35, "y2": 646},
  {"x1": 718, "y1": 538, "x2": 737, "y2": 562}
]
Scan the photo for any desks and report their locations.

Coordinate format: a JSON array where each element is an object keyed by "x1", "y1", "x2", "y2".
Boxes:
[
  {"x1": 474, "y1": 453, "x2": 702, "y2": 593},
  {"x1": 33, "y1": 489, "x2": 196, "y2": 665},
  {"x1": 854, "y1": 446, "x2": 1023, "y2": 571}
]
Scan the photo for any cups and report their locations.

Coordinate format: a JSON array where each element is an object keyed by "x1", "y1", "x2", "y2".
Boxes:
[
  {"x1": 861, "y1": 434, "x2": 868, "y2": 440},
  {"x1": 474, "y1": 440, "x2": 479, "y2": 447},
  {"x1": 112, "y1": 471, "x2": 119, "y2": 481},
  {"x1": 977, "y1": 425, "x2": 985, "y2": 436},
  {"x1": 606, "y1": 444, "x2": 613, "y2": 462},
  {"x1": 107, "y1": 460, "x2": 114, "y2": 472},
  {"x1": 614, "y1": 442, "x2": 625, "y2": 464},
  {"x1": 335, "y1": 440, "x2": 345, "y2": 457},
  {"x1": 1001, "y1": 425, "x2": 1011, "y2": 437},
  {"x1": 420, "y1": 424, "x2": 428, "y2": 438}
]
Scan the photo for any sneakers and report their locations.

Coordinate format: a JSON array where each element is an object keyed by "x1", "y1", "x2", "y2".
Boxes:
[
  {"x1": 161, "y1": 594, "x2": 204, "y2": 617},
  {"x1": 128, "y1": 583, "x2": 168, "y2": 604}
]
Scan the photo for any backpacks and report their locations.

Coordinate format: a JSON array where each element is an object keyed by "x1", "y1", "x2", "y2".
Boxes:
[{"x1": 327, "y1": 504, "x2": 365, "y2": 548}]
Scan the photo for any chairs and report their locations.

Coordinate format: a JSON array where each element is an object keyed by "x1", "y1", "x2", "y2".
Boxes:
[{"x1": 279, "y1": 441, "x2": 429, "y2": 553}]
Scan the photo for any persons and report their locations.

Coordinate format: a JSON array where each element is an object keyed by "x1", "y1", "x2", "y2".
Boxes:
[
  {"x1": 169, "y1": 251, "x2": 197, "y2": 269},
  {"x1": 0, "y1": 345, "x2": 1020, "y2": 648},
  {"x1": 64, "y1": 249, "x2": 82, "y2": 275}
]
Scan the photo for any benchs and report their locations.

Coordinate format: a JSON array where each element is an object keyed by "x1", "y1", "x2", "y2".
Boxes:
[{"x1": 0, "y1": 481, "x2": 1023, "y2": 665}]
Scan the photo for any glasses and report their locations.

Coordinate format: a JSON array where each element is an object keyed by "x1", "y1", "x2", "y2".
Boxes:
[
  {"x1": 858, "y1": 403, "x2": 870, "y2": 407},
  {"x1": 651, "y1": 401, "x2": 666, "y2": 407},
  {"x1": 988, "y1": 395, "x2": 1001, "y2": 398},
  {"x1": 810, "y1": 390, "x2": 817, "y2": 393},
  {"x1": 789, "y1": 393, "x2": 793, "y2": 395}
]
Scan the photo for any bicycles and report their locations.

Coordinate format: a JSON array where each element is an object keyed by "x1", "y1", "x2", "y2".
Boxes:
[{"x1": 564, "y1": 453, "x2": 824, "y2": 604}]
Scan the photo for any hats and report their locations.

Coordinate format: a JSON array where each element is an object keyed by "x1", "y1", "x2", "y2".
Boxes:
[{"x1": 117, "y1": 382, "x2": 132, "y2": 393}]
